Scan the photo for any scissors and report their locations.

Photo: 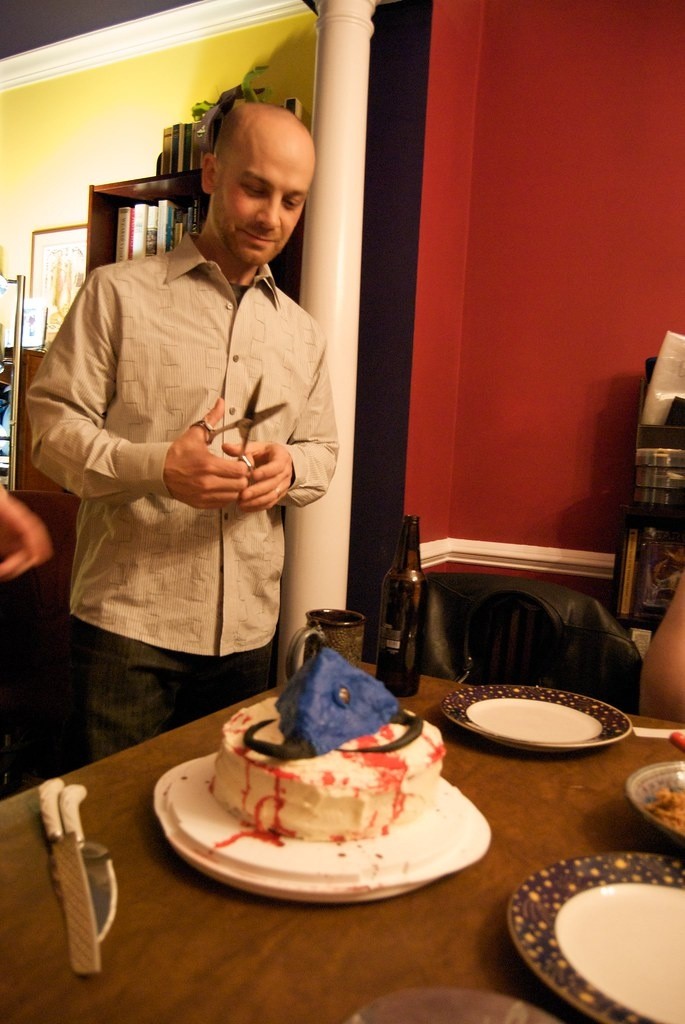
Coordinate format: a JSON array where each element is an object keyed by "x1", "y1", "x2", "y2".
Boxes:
[{"x1": 190, "y1": 374, "x2": 289, "y2": 475}]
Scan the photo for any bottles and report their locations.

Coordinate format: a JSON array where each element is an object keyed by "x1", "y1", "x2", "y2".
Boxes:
[{"x1": 375, "y1": 516, "x2": 428, "y2": 697}]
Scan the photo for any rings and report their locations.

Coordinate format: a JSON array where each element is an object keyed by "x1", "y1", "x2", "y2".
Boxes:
[{"x1": 274, "y1": 488, "x2": 281, "y2": 498}]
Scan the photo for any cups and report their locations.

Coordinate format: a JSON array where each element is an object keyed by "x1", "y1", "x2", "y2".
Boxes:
[{"x1": 286, "y1": 609, "x2": 366, "y2": 680}]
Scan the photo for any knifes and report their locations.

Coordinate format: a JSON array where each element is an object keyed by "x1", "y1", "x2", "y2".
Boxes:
[{"x1": 38, "y1": 777, "x2": 118, "y2": 975}]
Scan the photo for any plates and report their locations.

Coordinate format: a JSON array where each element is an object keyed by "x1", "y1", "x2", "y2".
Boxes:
[
  {"x1": 150, "y1": 752, "x2": 494, "y2": 906},
  {"x1": 625, "y1": 761, "x2": 685, "y2": 846},
  {"x1": 507, "y1": 851, "x2": 685, "y2": 1024},
  {"x1": 440, "y1": 685, "x2": 633, "y2": 754}
]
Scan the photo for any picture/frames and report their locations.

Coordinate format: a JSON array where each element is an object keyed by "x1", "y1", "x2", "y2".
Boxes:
[{"x1": 27, "y1": 222, "x2": 88, "y2": 352}]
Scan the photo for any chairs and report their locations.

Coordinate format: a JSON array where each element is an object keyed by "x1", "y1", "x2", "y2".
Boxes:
[
  {"x1": 0, "y1": 488, "x2": 82, "y2": 780},
  {"x1": 411, "y1": 571, "x2": 644, "y2": 715}
]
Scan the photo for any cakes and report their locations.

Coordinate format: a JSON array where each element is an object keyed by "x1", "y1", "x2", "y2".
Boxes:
[{"x1": 211, "y1": 648, "x2": 446, "y2": 843}]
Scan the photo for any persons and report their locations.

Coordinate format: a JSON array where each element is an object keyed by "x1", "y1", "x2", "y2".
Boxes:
[
  {"x1": 0, "y1": 478, "x2": 58, "y2": 594},
  {"x1": 28, "y1": 103, "x2": 343, "y2": 769},
  {"x1": 637, "y1": 569, "x2": 685, "y2": 722}
]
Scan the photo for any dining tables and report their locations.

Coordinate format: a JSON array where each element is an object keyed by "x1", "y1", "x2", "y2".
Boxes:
[{"x1": 0, "y1": 661, "x2": 685, "y2": 1024}]
